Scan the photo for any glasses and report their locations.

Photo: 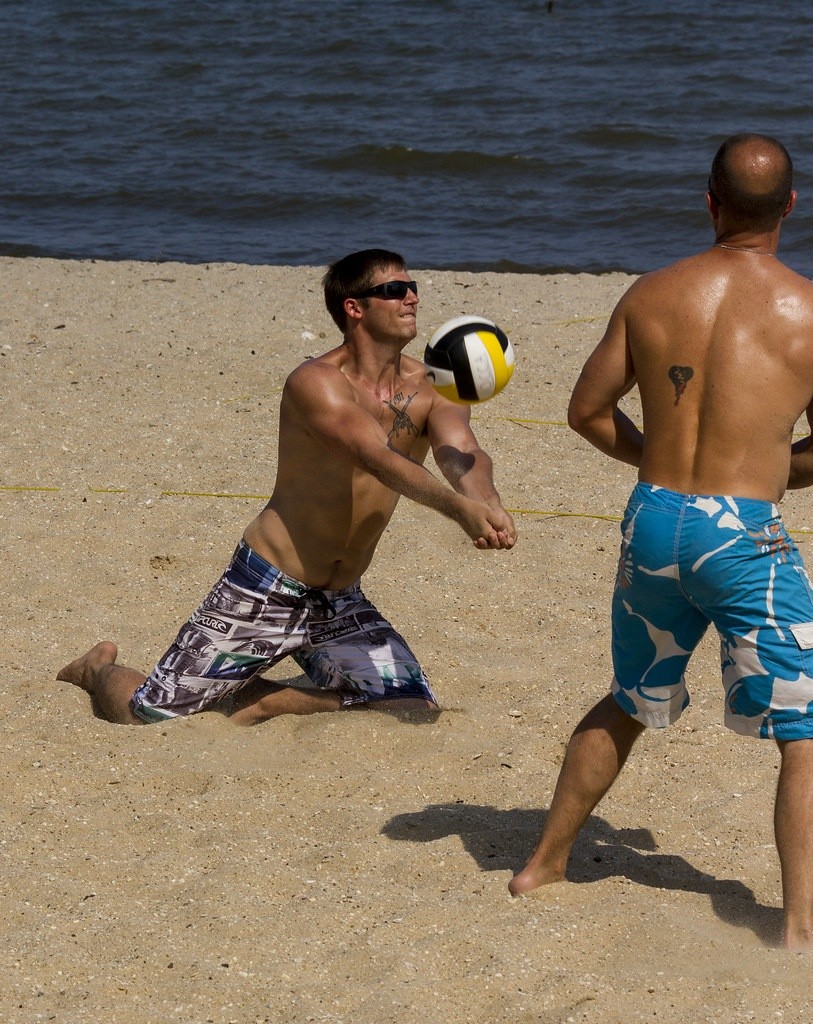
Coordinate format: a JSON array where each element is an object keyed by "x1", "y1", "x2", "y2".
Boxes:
[{"x1": 339, "y1": 281, "x2": 417, "y2": 315}]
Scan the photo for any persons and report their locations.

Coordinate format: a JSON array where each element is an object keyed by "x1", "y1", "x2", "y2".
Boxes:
[
  {"x1": 55, "y1": 248, "x2": 519, "y2": 726},
  {"x1": 508, "y1": 133, "x2": 813, "y2": 951}
]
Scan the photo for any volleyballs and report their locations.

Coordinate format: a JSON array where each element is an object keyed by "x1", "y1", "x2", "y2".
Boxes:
[{"x1": 423, "y1": 314, "x2": 516, "y2": 406}]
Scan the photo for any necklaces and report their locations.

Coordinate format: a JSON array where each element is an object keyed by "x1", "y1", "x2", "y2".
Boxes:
[{"x1": 712, "y1": 244, "x2": 775, "y2": 260}]
